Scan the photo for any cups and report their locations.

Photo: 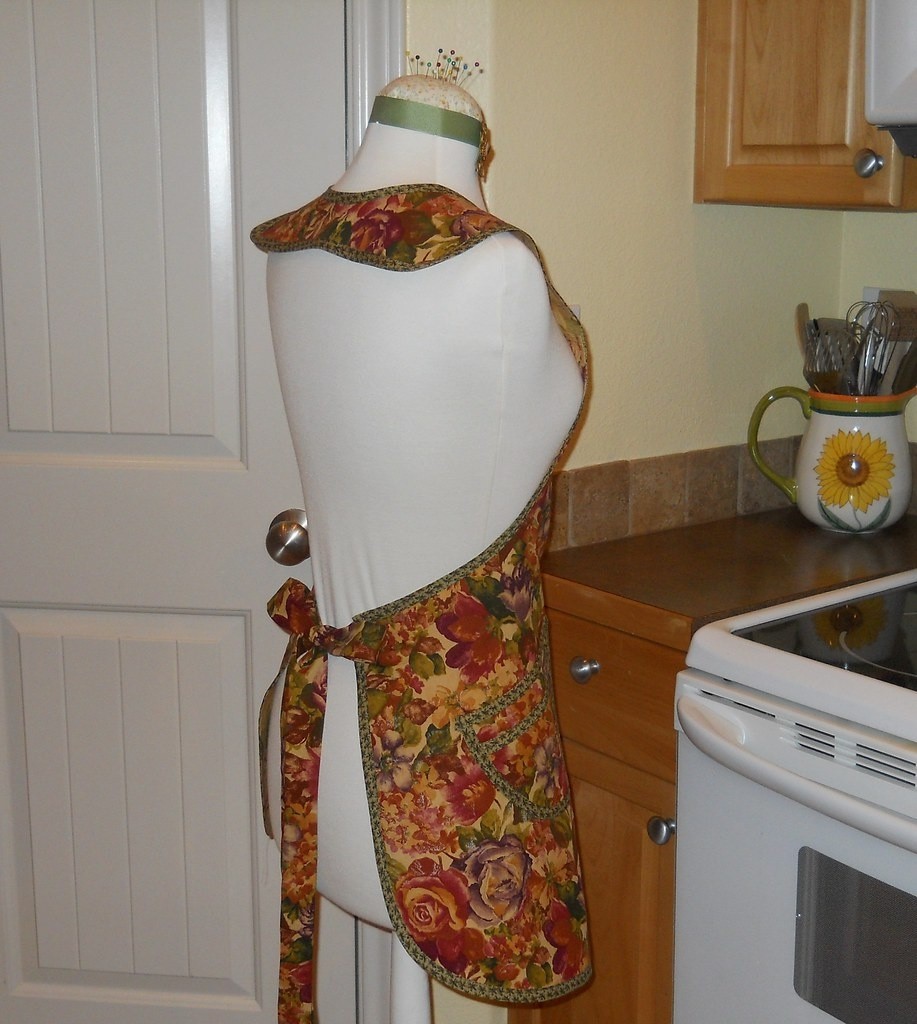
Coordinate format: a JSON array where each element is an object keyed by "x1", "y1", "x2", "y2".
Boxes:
[{"x1": 748, "y1": 385, "x2": 917, "y2": 535}]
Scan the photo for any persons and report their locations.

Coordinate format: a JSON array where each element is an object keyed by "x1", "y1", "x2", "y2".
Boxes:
[{"x1": 248, "y1": 72, "x2": 594, "y2": 1023}]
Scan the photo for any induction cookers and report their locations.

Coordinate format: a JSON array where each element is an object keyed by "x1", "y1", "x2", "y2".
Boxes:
[{"x1": 686, "y1": 567, "x2": 917, "y2": 745}]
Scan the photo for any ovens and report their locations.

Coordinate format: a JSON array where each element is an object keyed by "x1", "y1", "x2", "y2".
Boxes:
[{"x1": 672, "y1": 670, "x2": 917, "y2": 1024}]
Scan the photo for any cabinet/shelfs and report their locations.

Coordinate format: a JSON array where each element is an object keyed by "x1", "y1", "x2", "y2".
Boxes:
[
  {"x1": 692, "y1": 0, "x2": 917, "y2": 214},
  {"x1": 508, "y1": 505, "x2": 917, "y2": 1024}
]
